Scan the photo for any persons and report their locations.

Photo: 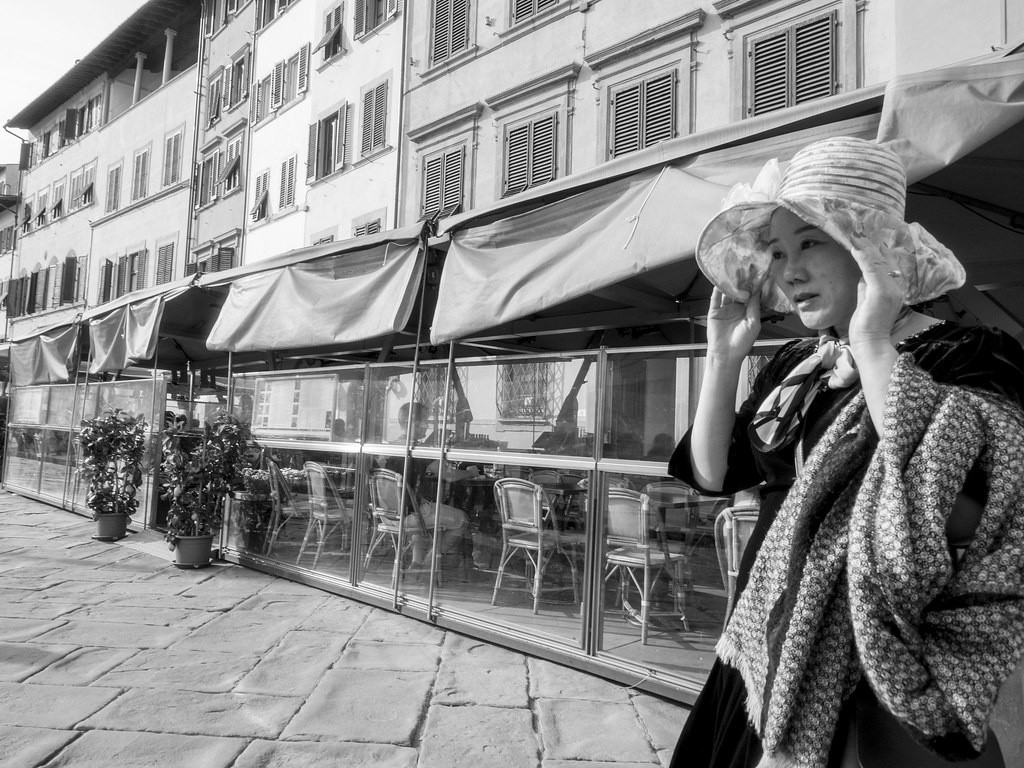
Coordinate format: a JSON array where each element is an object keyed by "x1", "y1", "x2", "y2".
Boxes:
[
  {"x1": 666, "y1": 135, "x2": 1024, "y2": 768},
  {"x1": 157, "y1": 392, "x2": 252, "y2": 438},
  {"x1": 383, "y1": 402, "x2": 480, "y2": 586},
  {"x1": 532, "y1": 398, "x2": 676, "y2": 462},
  {"x1": 327, "y1": 418, "x2": 353, "y2": 442}
]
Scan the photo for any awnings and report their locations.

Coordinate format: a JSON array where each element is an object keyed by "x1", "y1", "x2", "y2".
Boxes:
[
  {"x1": 7, "y1": 49, "x2": 1022, "y2": 711},
  {"x1": 4, "y1": 0, "x2": 205, "y2": 128}
]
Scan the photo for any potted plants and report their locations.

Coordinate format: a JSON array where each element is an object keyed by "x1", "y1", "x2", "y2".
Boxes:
[
  {"x1": 75, "y1": 409, "x2": 148, "y2": 540},
  {"x1": 158, "y1": 411, "x2": 254, "y2": 568}
]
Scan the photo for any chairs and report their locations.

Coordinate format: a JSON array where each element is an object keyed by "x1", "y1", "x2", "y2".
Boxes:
[{"x1": 262, "y1": 457, "x2": 764, "y2": 646}]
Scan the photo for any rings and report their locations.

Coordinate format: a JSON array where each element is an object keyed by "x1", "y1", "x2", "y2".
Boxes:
[{"x1": 888, "y1": 269, "x2": 901, "y2": 279}]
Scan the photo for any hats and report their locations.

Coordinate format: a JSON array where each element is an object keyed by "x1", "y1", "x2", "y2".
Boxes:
[{"x1": 695, "y1": 136, "x2": 967, "y2": 313}]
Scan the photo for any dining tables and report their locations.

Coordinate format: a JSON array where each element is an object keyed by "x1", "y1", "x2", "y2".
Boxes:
[
  {"x1": 420, "y1": 475, "x2": 502, "y2": 583},
  {"x1": 322, "y1": 463, "x2": 356, "y2": 560},
  {"x1": 527, "y1": 474, "x2": 586, "y2": 606},
  {"x1": 624, "y1": 487, "x2": 730, "y2": 632}
]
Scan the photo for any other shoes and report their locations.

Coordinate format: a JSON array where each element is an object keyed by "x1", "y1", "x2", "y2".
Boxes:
[{"x1": 408, "y1": 562, "x2": 430, "y2": 586}]
